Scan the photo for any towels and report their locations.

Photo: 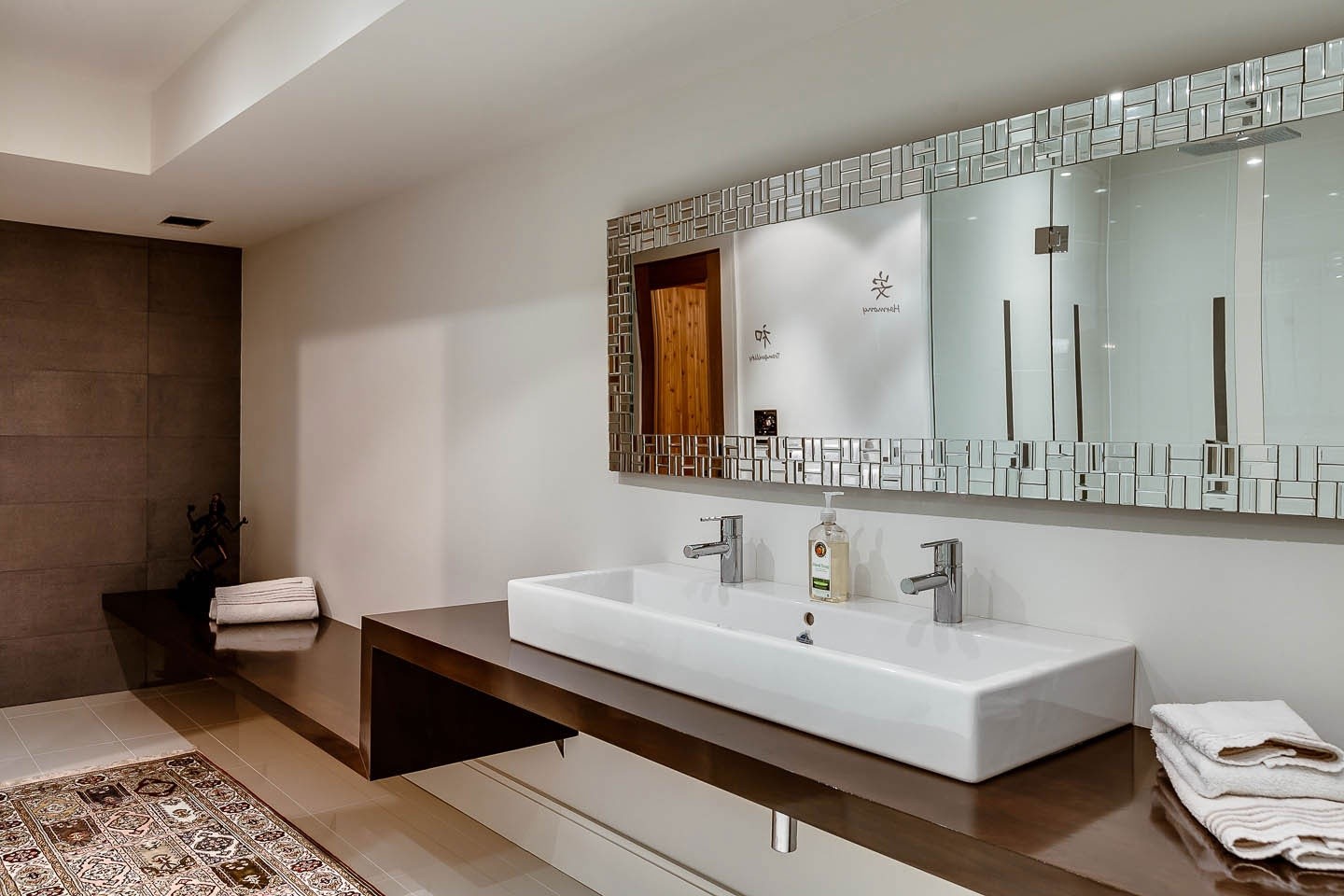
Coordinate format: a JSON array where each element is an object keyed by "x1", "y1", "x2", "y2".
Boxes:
[
  {"x1": 1146, "y1": 698, "x2": 1344, "y2": 872},
  {"x1": 210, "y1": 620, "x2": 317, "y2": 651},
  {"x1": 1152, "y1": 769, "x2": 1343, "y2": 895},
  {"x1": 207, "y1": 574, "x2": 321, "y2": 628}
]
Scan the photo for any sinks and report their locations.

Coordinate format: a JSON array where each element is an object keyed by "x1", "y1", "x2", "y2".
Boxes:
[{"x1": 506, "y1": 564, "x2": 1138, "y2": 786}]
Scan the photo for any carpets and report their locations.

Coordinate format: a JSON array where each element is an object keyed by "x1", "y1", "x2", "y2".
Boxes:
[{"x1": 0, "y1": 745, "x2": 388, "y2": 896}]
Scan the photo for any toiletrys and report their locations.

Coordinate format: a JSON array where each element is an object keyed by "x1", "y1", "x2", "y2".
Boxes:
[{"x1": 807, "y1": 491, "x2": 852, "y2": 605}]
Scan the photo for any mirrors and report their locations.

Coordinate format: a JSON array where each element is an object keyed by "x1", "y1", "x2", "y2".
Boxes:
[{"x1": 606, "y1": 32, "x2": 1344, "y2": 521}]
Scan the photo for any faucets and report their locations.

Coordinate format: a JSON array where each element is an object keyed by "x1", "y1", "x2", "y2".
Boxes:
[
  {"x1": 683, "y1": 513, "x2": 744, "y2": 583},
  {"x1": 900, "y1": 538, "x2": 965, "y2": 626}
]
